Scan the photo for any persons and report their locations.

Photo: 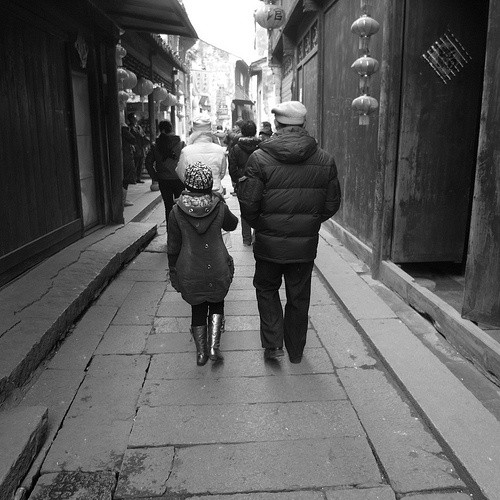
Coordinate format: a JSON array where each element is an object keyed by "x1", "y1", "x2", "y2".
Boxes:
[
  {"x1": 229, "y1": 121, "x2": 262, "y2": 245},
  {"x1": 213, "y1": 125, "x2": 226, "y2": 147},
  {"x1": 146, "y1": 121, "x2": 184, "y2": 245},
  {"x1": 175, "y1": 114, "x2": 233, "y2": 252},
  {"x1": 166, "y1": 162, "x2": 238, "y2": 366},
  {"x1": 227, "y1": 119, "x2": 245, "y2": 195},
  {"x1": 122, "y1": 113, "x2": 152, "y2": 184},
  {"x1": 259, "y1": 122, "x2": 272, "y2": 141},
  {"x1": 240, "y1": 101, "x2": 341, "y2": 363}
]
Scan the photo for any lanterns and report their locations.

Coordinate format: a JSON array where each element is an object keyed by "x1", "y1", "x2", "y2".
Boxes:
[
  {"x1": 350, "y1": 55, "x2": 380, "y2": 91},
  {"x1": 117, "y1": 44, "x2": 128, "y2": 109},
  {"x1": 125, "y1": 68, "x2": 177, "y2": 113},
  {"x1": 351, "y1": 94, "x2": 379, "y2": 125},
  {"x1": 352, "y1": 14, "x2": 379, "y2": 49},
  {"x1": 255, "y1": 4, "x2": 286, "y2": 30}
]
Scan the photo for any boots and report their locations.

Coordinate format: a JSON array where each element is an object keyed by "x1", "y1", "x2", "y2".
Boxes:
[
  {"x1": 209, "y1": 314, "x2": 225, "y2": 361},
  {"x1": 122, "y1": 187, "x2": 133, "y2": 205},
  {"x1": 188, "y1": 324, "x2": 209, "y2": 366}
]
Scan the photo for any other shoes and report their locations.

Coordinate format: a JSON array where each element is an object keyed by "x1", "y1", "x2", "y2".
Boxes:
[
  {"x1": 243, "y1": 239, "x2": 250, "y2": 245},
  {"x1": 263, "y1": 347, "x2": 284, "y2": 359}
]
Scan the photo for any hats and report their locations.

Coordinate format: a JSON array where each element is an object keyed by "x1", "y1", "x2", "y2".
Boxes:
[
  {"x1": 259, "y1": 127, "x2": 273, "y2": 136},
  {"x1": 193, "y1": 114, "x2": 211, "y2": 133},
  {"x1": 271, "y1": 100, "x2": 307, "y2": 125},
  {"x1": 185, "y1": 160, "x2": 213, "y2": 193}
]
